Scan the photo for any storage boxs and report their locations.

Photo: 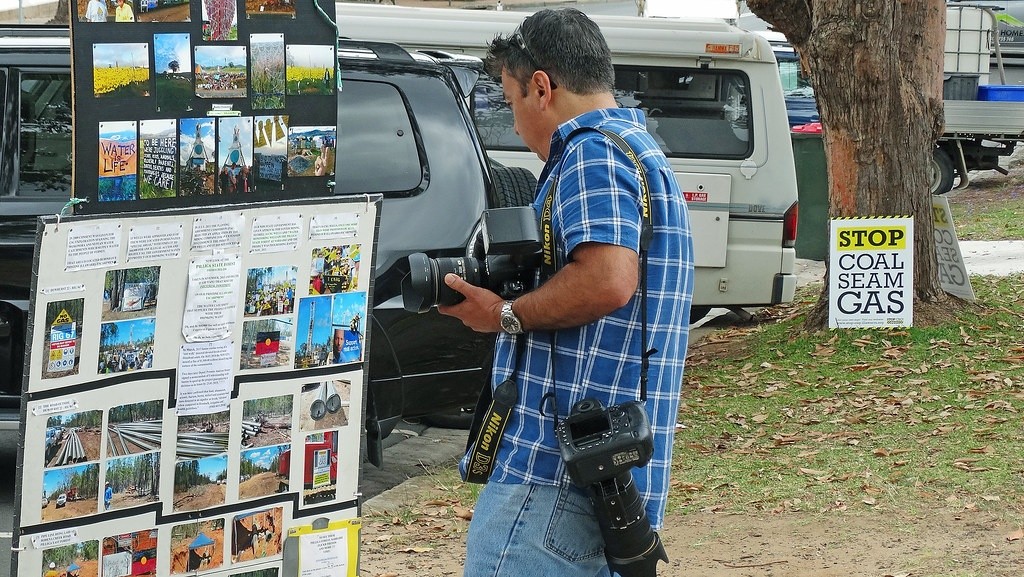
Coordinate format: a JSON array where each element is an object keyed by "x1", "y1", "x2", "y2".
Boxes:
[
  {"x1": 943, "y1": 74, "x2": 980, "y2": 100},
  {"x1": 978, "y1": 85, "x2": 1024, "y2": 102}
]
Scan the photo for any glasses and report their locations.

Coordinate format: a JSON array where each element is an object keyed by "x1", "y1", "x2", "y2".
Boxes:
[{"x1": 508, "y1": 16, "x2": 557, "y2": 90}]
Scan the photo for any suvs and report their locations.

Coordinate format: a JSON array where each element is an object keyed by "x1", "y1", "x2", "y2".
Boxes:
[
  {"x1": 1, "y1": 39, "x2": 538, "y2": 471},
  {"x1": 56, "y1": 493, "x2": 68, "y2": 509}
]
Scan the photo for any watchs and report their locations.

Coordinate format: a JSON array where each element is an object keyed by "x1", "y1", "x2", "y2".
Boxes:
[{"x1": 500, "y1": 301, "x2": 526, "y2": 335}]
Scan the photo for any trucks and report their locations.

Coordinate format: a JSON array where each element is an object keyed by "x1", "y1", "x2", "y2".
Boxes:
[{"x1": 753, "y1": 31, "x2": 1024, "y2": 195}]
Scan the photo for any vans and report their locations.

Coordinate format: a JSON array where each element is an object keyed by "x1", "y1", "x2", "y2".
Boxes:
[{"x1": 337, "y1": 0, "x2": 798, "y2": 326}]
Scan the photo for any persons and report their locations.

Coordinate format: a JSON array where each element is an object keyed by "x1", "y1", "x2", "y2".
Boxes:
[
  {"x1": 437, "y1": 10, "x2": 694, "y2": 577},
  {"x1": 85, "y1": 2, "x2": 331, "y2": 195},
  {"x1": 99, "y1": 249, "x2": 355, "y2": 573}
]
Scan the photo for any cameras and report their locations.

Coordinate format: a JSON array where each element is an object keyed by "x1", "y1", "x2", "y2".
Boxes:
[
  {"x1": 401, "y1": 206, "x2": 545, "y2": 314},
  {"x1": 553, "y1": 396, "x2": 669, "y2": 577}
]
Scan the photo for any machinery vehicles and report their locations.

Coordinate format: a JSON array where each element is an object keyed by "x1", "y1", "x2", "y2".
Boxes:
[{"x1": 67, "y1": 486, "x2": 78, "y2": 502}]
